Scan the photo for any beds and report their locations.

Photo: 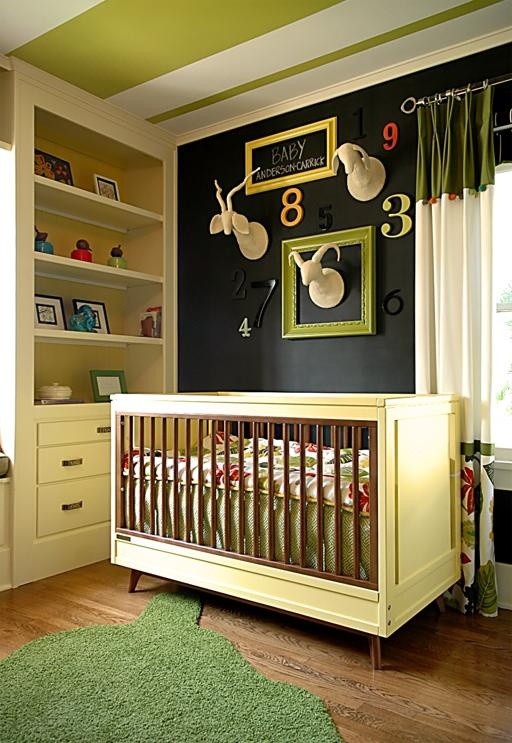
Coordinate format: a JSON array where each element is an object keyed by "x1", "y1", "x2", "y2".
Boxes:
[{"x1": 111, "y1": 391, "x2": 462, "y2": 670}]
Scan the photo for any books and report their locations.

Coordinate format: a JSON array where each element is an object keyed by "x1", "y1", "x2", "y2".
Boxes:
[
  {"x1": 136, "y1": 305, "x2": 162, "y2": 337},
  {"x1": 34, "y1": 399, "x2": 86, "y2": 405}
]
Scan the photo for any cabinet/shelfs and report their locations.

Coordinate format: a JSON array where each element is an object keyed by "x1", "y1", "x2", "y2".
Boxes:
[{"x1": 1, "y1": 55, "x2": 176, "y2": 589}]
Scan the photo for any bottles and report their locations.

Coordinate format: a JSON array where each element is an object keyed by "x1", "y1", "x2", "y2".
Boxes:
[
  {"x1": 71, "y1": 250, "x2": 92, "y2": 262},
  {"x1": 34, "y1": 240, "x2": 53, "y2": 253},
  {"x1": 107, "y1": 257, "x2": 127, "y2": 269}
]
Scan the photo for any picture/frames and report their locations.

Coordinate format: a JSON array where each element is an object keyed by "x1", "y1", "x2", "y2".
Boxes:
[
  {"x1": 35, "y1": 145, "x2": 121, "y2": 201},
  {"x1": 36, "y1": 294, "x2": 127, "y2": 402},
  {"x1": 244, "y1": 115, "x2": 377, "y2": 338}
]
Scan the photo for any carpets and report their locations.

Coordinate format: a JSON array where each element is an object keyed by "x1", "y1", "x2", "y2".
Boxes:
[{"x1": 2, "y1": 589, "x2": 344, "y2": 739}]
